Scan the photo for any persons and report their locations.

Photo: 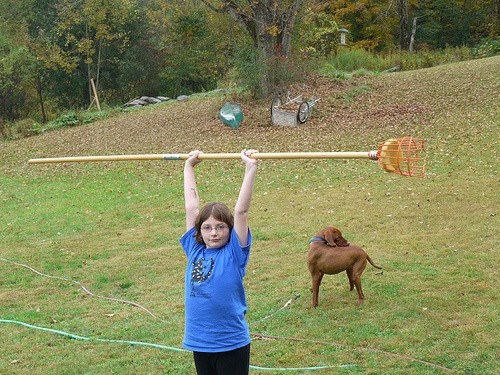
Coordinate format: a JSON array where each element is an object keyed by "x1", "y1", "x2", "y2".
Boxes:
[{"x1": 179, "y1": 149, "x2": 258, "y2": 375}]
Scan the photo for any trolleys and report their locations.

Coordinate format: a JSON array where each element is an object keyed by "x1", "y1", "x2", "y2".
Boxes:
[{"x1": 270, "y1": 93, "x2": 323, "y2": 128}]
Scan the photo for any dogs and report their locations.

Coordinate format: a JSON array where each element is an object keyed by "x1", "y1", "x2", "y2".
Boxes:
[{"x1": 307, "y1": 226, "x2": 382, "y2": 309}]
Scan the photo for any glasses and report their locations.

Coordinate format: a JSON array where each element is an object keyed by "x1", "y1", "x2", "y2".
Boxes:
[{"x1": 201, "y1": 224, "x2": 229, "y2": 232}]
